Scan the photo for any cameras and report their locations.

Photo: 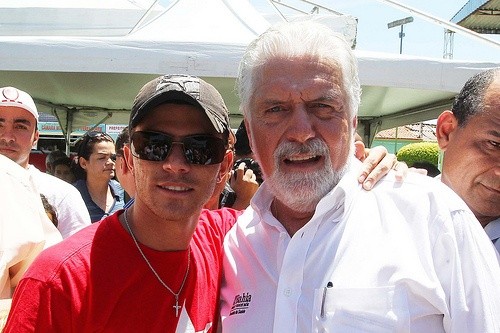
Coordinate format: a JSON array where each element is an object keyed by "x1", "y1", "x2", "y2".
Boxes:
[{"x1": 233, "y1": 158, "x2": 264, "y2": 184}]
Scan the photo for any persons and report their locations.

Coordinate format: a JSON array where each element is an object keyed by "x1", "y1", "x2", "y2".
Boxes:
[
  {"x1": 432, "y1": 67, "x2": 500, "y2": 256},
  {"x1": 215, "y1": 22, "x2": 500, "y2": 333},
  {"x1": 114, "y1": 126, "x2": 135, "y2": 209},
  {"x1": 204, "y1": 118, "x2": 264, "y2": 210},
  {"x1": 71, "y1": 131, "x2": 125, "y2": 224},
  {"x1": 0, "y1": 86, "x2": 91, "y2": 241},
  {"x1": 0, "y1": 74, "x2": 408, "y2": 333},
  {"x1": 45, "y1": 150, "x2": 77, "y2": 184}
]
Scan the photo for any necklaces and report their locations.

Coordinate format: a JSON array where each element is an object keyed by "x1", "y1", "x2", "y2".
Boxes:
[{"x1": 123, "y1": 208, "x2": 191, "y2": 318}]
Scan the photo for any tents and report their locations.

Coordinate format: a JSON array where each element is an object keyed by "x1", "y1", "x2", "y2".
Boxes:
[{"x1": 0, "y1": 0, "x2": 500, "y2": 158}]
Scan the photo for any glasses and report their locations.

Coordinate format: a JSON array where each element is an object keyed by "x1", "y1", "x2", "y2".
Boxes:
[
  {"x1": 131, "y1": 131, "x2": 224, "y2": 164},
  {"x1": 85, "y1": 132, "x2": 114, "y2": 145}
]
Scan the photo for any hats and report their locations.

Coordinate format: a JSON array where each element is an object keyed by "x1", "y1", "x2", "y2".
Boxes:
[
  {"x1": 0, "y1": 87, "x2": 38, "y2": 125},
  {"x1": 129, "y1": 74, "x2": 229, "y2": 133}
]
